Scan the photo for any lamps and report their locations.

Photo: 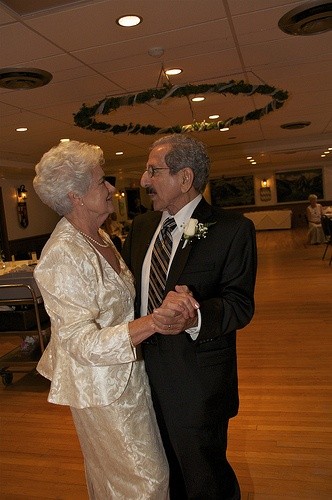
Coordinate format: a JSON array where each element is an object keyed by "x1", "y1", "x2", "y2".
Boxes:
[
  {"x1": 259, "y1": 176, "x2": 272, "y2": 201},
  {"x1": 16, "y1": 185, "x2": 29, "y2": 227},
  {"x1": 115, "y1": 191, "x2": 126, "y2": 215}
]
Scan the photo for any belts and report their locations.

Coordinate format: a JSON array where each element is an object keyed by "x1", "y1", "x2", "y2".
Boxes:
[{"x1": 142, "y1": 338, "x2": 156, "y2": 345}]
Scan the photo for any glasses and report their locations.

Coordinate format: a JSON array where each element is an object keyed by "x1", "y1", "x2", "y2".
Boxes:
[{"x1": 143, "y1": 163, "x2": 173, "y2": 178}]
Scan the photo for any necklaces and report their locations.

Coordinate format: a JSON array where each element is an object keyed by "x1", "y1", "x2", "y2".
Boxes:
[{"x1": 80, "y1": 231, "x2": 110, "y2": 248}]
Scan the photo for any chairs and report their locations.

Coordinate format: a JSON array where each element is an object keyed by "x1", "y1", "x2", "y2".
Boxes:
[{"x1": 320, "y1": 214, "x2": 332, "y2": 265}]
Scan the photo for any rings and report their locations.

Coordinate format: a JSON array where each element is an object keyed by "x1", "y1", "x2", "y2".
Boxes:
[{"x1": 168, "y1": 324, "x2": 171, "y2": 329}]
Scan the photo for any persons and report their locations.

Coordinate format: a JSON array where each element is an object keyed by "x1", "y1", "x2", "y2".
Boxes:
[
  {"x1": 100, "y1": 205, "x2": 148, "y2": 251},
  {"x1": 121, "y1": 132, "x2": 258, "y2": 500},
  {"x1": 32, "y1": 141, "x2": 200, "y2": 500},
  {"x1": 305, "y1": 194, "x2": 328, "y2": 244}
]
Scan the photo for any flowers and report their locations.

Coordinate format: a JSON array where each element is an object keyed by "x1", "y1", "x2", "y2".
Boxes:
[{"x1": 180, "y1": 218, "x2": 217, "y2": 249}]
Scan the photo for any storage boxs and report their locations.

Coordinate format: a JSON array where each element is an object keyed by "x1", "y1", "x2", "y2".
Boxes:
[{"x1": 0, "y1": 260, "x2": 41, "y2": 298}]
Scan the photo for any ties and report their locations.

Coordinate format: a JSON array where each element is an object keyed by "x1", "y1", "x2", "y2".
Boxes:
[{"x1": 147, "y1": 218, "x2": 177, "y2": 315}]
